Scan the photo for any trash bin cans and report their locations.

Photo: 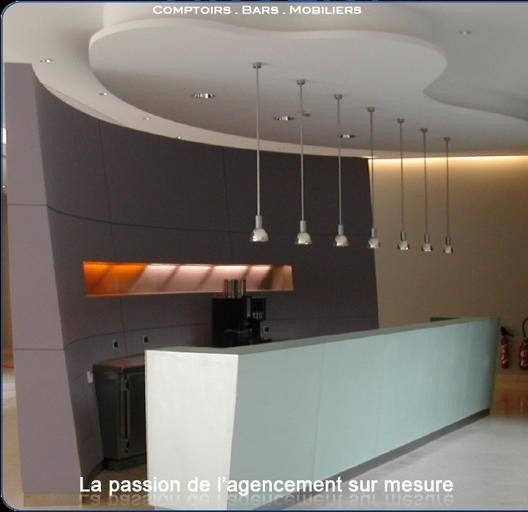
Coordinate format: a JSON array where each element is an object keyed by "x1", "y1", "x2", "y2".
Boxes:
[{"x1": 92, "y1": 353, "x2": 147, "y2": 471}]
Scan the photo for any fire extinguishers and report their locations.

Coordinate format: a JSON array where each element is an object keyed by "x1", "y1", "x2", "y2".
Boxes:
[{"x1": 500, "y1": 318, "x2": 528, "y2": 369}]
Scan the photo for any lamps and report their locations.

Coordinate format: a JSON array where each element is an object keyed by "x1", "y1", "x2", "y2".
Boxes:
[{"x1": 248, "y1": 61, "x2": 454, "y2": 257}]
